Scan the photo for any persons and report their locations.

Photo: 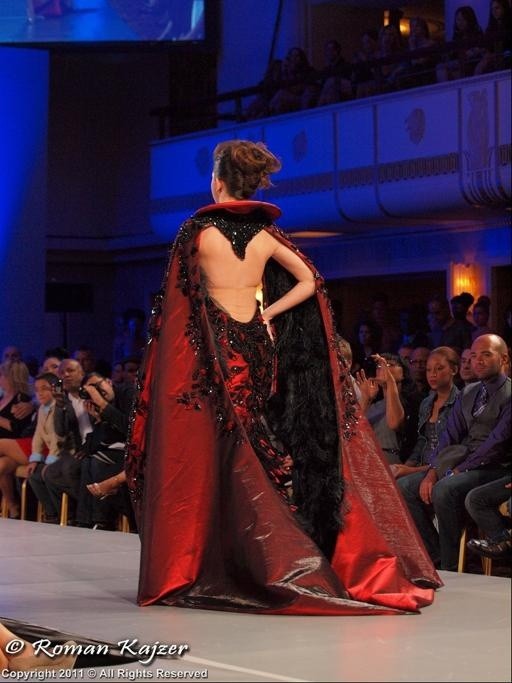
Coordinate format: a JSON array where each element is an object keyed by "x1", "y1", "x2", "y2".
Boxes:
[
  {"x1": 1, "y1": 618, "x2": 79, "y2": 672},
  {"x1": 242, "y1": 0, "x2": 509, "y2": 120},
  {"x1": 172, "y1": 134, "x2": 320, "y2": 605},
  {"x1": 0, "y1": 287, "x2": 512, "y2": 579}
]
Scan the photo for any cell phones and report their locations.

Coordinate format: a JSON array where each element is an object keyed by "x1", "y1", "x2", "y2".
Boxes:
[
  {"x1": 55, "y1": 379, "x2": 63, "y2": 392},
  {"x1": 365, "y1": 356, "x2": 376, "y2": 378}
]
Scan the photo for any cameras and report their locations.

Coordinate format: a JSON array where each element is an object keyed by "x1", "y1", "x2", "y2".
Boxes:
[{"x1": 78, "y1": 387, "x2": 100, "y2": 412}]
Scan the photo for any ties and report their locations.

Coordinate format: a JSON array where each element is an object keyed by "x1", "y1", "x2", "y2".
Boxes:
[{"x1": 473, "y1": 385, "x2": 488, "y2": 418}]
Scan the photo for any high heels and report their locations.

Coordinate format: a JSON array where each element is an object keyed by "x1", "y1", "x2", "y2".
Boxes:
[
  {"x1": 9, "y1": 640, "x2": 78, "y2": 671},
  {"x1": 87, "y1": 483, "x2": 118, "y2": 500}
]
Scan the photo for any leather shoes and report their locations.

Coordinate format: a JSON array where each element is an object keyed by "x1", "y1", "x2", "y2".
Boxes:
[{"x1": 467, "y1": 529, "x2": 512, "y2": 560}]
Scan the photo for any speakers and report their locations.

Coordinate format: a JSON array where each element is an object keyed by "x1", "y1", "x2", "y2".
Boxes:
[{"x1": 47, "y1": 269, "x2": 94, "y2": 313}]
